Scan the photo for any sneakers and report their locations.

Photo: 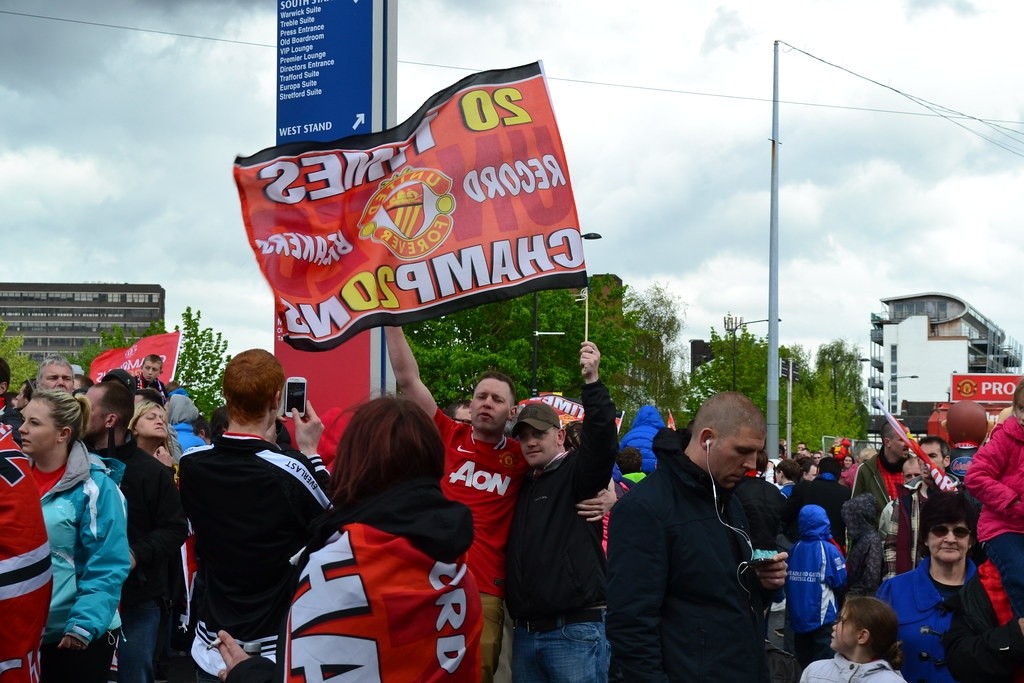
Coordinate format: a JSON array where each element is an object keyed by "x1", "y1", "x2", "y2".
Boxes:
[{"x1": 771, "y1": 599, "x2": 787, "y2": 612}]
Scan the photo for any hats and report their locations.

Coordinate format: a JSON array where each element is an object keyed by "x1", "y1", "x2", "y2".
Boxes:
[{"x1": 511, "y1": 402, "x2": 560, "y2": 439}]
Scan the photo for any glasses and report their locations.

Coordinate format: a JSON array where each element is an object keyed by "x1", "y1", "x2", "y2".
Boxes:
[
  {"x1": 931, "y1": 526, "x2": 971, "y2": 538},
  {"x1": 813, "y1": 457, "x2": 822, "y2": 459}
]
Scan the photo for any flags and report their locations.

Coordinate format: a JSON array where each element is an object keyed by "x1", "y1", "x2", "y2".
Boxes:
[
  {"x1": 874, "y1": 401, "x2": 956, "y2": 490},
  {"x1": 232, "y1": 60, "x2": 588, "y2": 352},
  {"x1": 89, "y1": 330, "x2": 181, "y2": 383}
]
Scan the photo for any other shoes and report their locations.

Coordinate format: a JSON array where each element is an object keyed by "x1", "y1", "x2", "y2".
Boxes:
[{"x1": 774, "y1": 629, "x2": 785, "y2": 636}]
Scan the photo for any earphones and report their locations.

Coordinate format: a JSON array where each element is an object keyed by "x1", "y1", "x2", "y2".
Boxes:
[{"x1": 706, "y1": 440, "x2": 711, "y2": 453}]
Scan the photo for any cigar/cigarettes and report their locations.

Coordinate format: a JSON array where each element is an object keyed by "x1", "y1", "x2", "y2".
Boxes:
[{"x1": 207, "y1": 638, "x2": 223, "y2": 650}]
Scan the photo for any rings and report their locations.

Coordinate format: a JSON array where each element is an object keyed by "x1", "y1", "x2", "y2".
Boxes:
[
  {"x1": 591, "y1": 348, "x2": 595, "y2": 353},
  {"x1": 76, "y1": 644, "x2": 81, "y2": 647},
  {"x1": 219, "y1": 671, "x2": 226, "y2": 679}
]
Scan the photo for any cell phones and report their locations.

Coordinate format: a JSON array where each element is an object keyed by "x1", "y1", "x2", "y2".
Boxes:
[
  {"x1": 285, "y1": 377, "x2": 307, "y2": 418},
  {"x1": 747, "y1": 558, "x2": 776, "y2": 566}
]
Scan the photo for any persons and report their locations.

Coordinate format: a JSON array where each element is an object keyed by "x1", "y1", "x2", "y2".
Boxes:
[{"x1": 0, "y1": 322, "x2": 1024, "y2": 683}]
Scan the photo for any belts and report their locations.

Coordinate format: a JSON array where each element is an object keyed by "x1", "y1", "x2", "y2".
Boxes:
[{"x1": 514, "y1": 608, "x2": 601, "y2": 632}]
